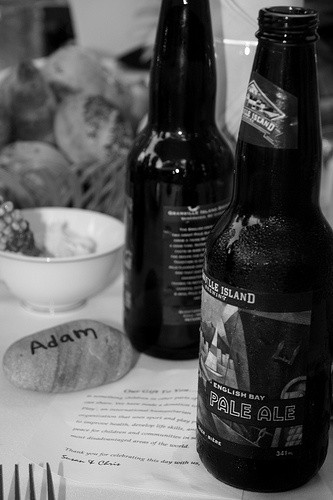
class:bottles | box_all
[197,5,333,494]
[125,0,233,360]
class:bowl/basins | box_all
[0,207,126,313]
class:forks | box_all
[0,462,55,500]
